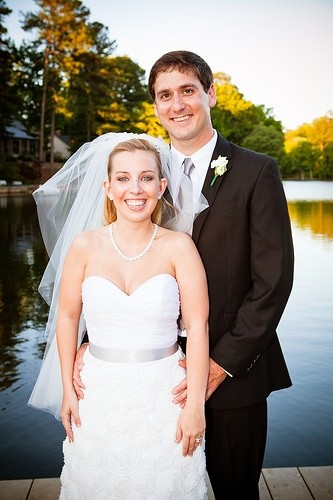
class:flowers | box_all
[211,155,228,186]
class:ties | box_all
[177,157,194,238]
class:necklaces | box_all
[110,222,158,261]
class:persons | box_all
[27,133,208,500]
[72,51,295,500]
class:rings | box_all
[196,438,201,443]
